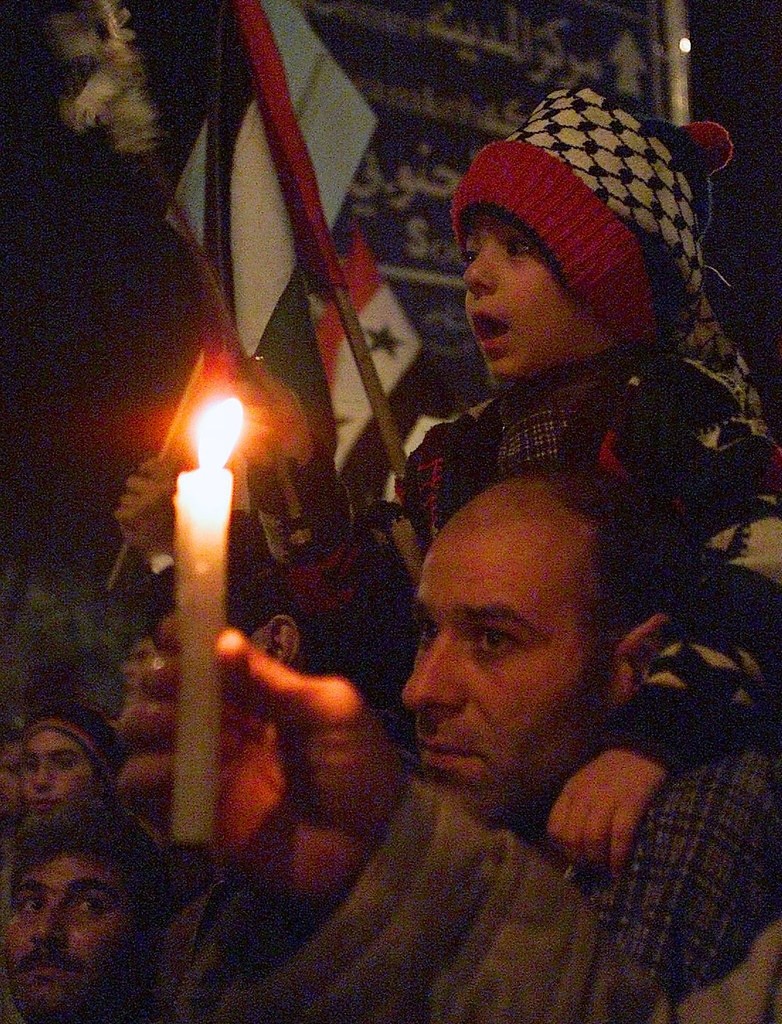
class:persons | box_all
[0,88,782,1024]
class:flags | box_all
[163,0,424,479]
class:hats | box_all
[25,699,111,766]
[452,88,732,337]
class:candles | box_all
[170,398,243,847]
